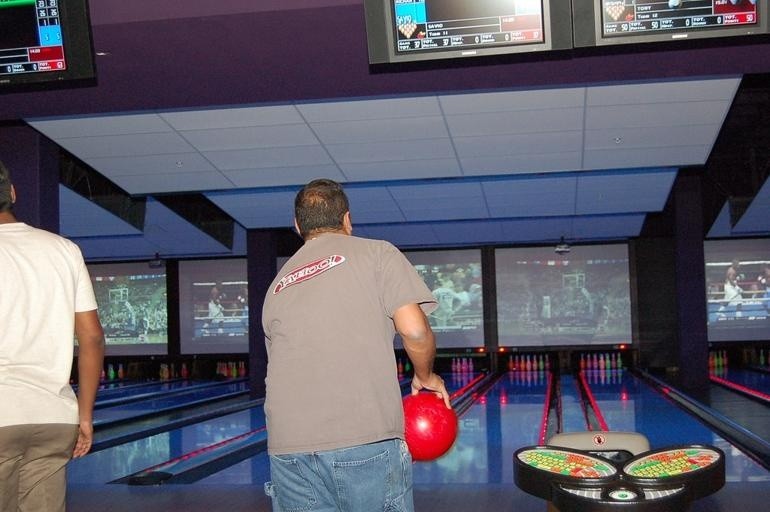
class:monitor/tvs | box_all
[1,0,99,93]
[363,0,572,65]
[572,0,770,49]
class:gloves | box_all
[736,274,745,281]
[219,293,227,300]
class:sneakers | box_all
[716,311,726,317]
[201,328,209,333]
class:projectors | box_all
[555,244,570,255]
[148,260,166,268]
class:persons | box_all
[416,263,482,326]
[0,160,106,511]
[717,260,743,319]
[706,271,768,299]
[92,272,249,341]
[260,177,452,512]
[495,273,632,332]
